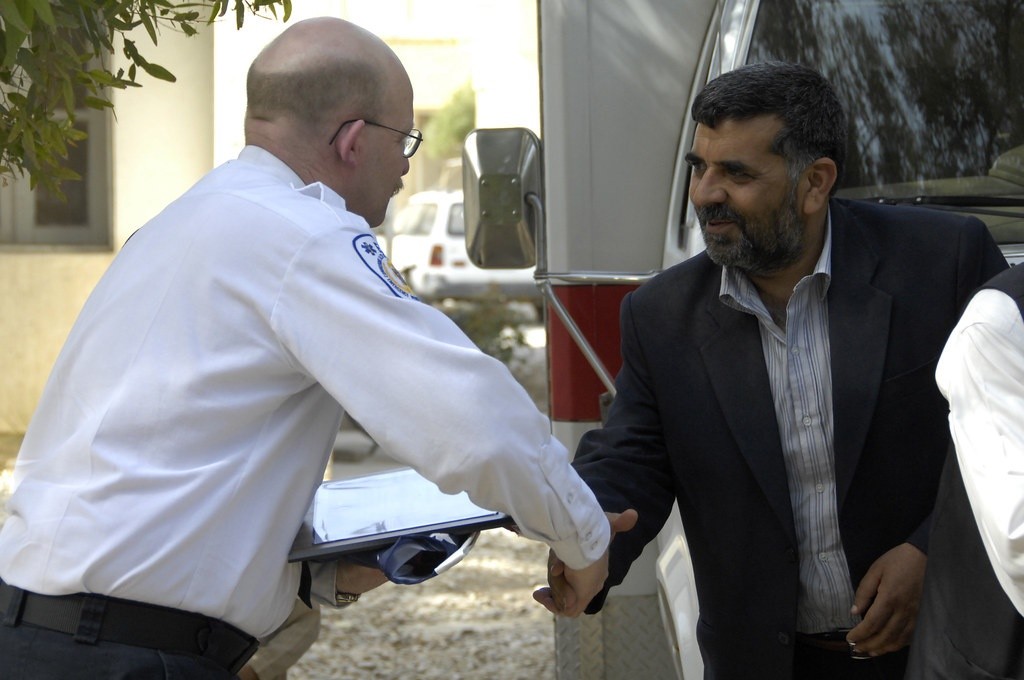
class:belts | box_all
[800,631,876,659]
[1,586,259,675]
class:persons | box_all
[906,261,1024,680]
[0,17,610,680]
[532,64,1011,680]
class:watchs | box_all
[334,588,363,604]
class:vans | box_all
[391,189,544,324]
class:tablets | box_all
[287,465,507,564]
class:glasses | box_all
[329,115,424,158]
